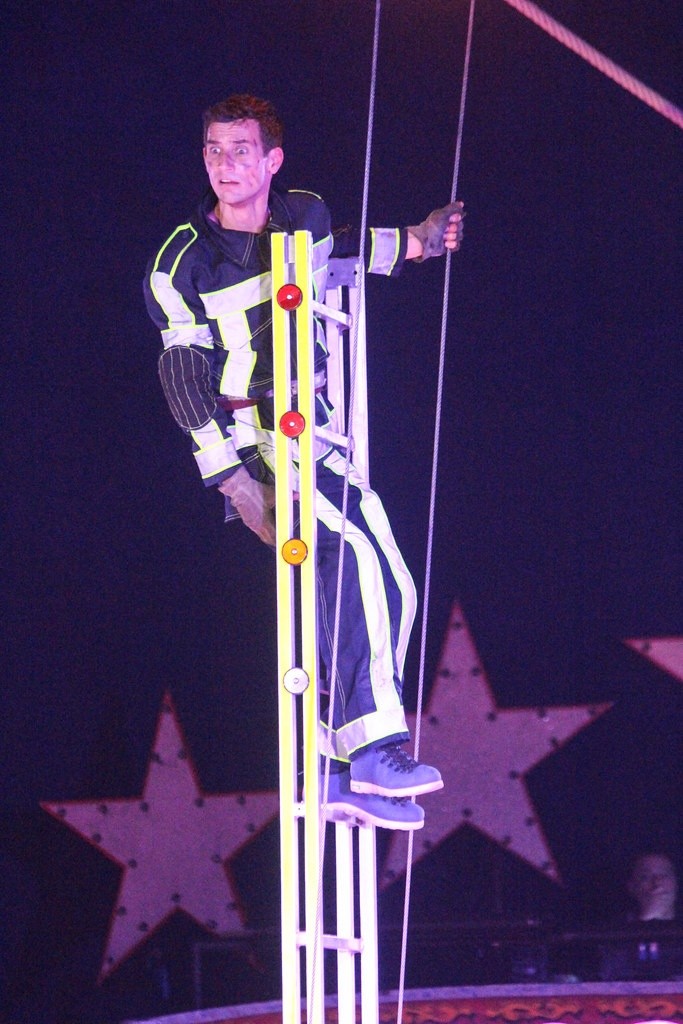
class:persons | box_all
[141,93,464,831]
[613,848,683,981]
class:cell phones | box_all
[653,872,672,893]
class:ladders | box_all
[270,229,380,1024]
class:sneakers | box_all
[350,743,444,798]
[302,765,425,830]
